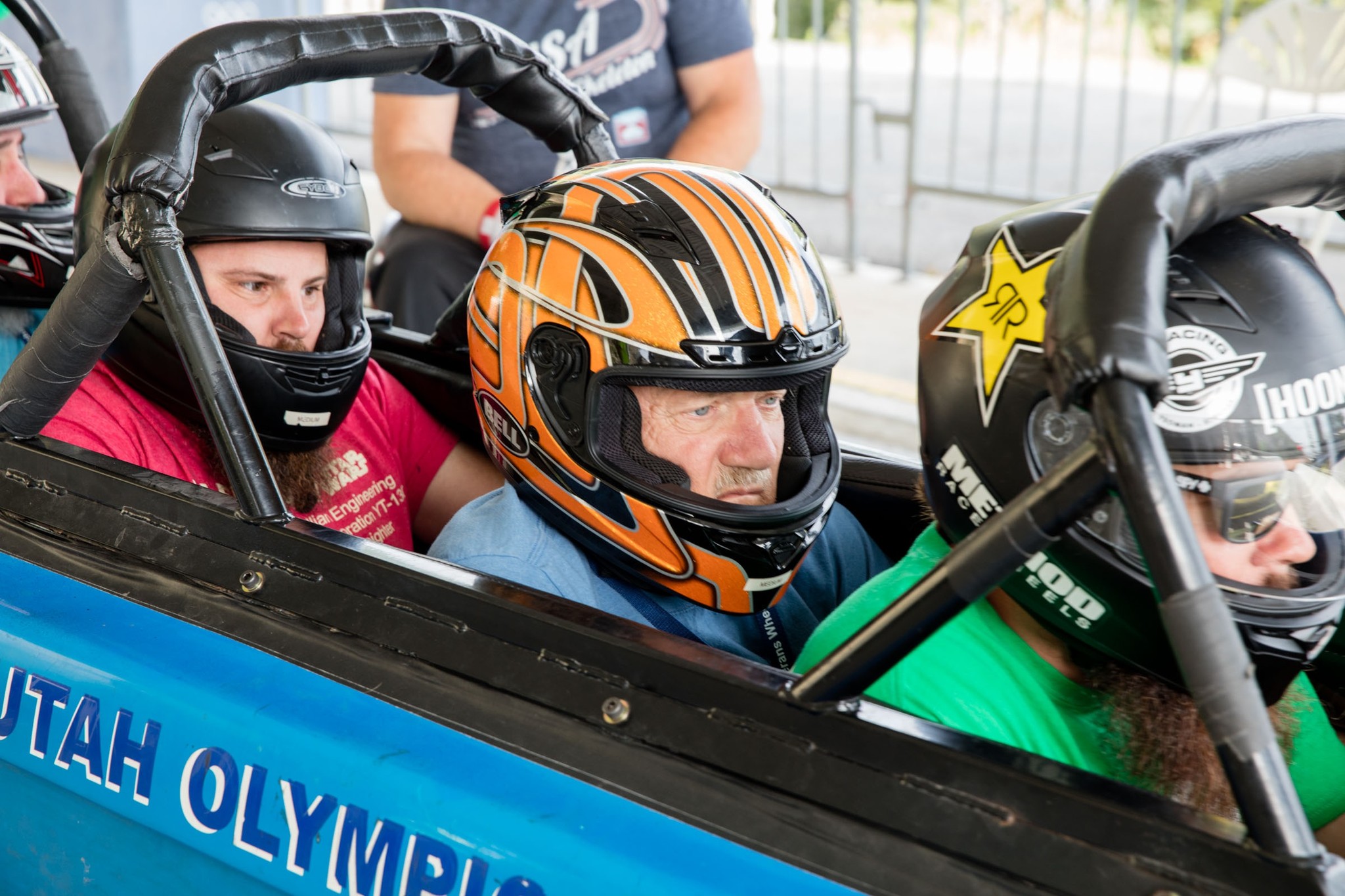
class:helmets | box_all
[70,94,374,451]
[466,158,849,611]
[2,35,80,308]
[916,183,1345,706]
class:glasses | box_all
[1171,449,1343,546]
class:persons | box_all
[0,34,1345,856]
[365,0,766,333]
